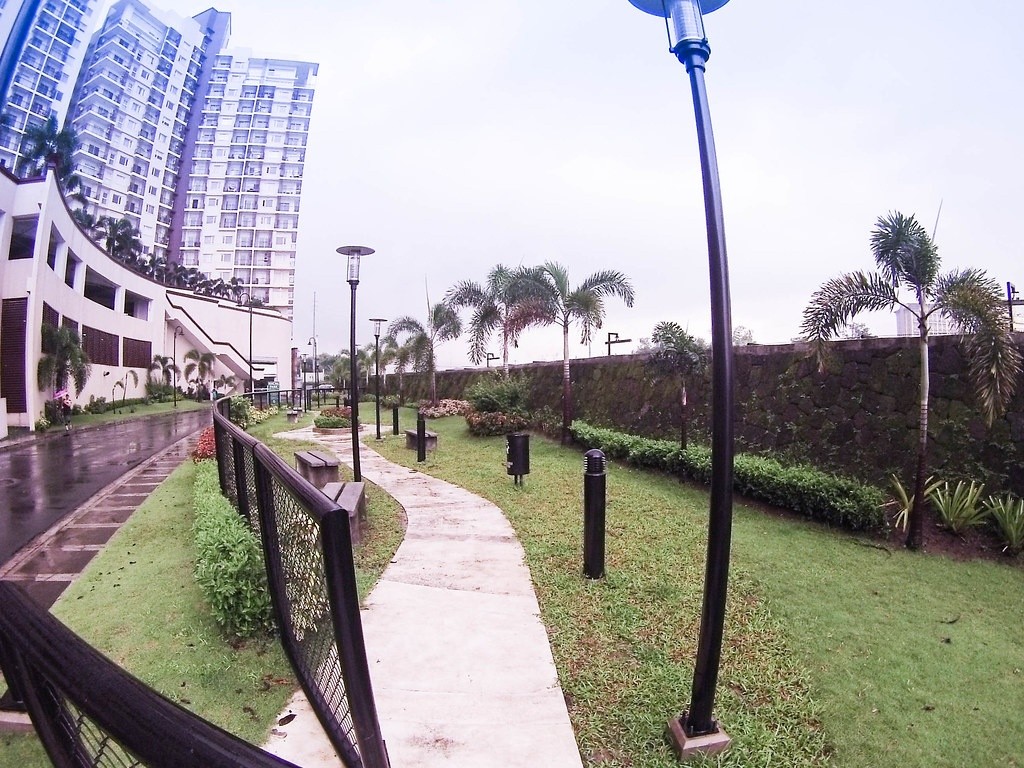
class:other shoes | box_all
[69,424,72,429]
[65,426,69,431]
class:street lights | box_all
[368,318,388,441]
[1001,282,1024,332]
[307,337,317,389]
[235,292,253,393]
[300,353,309,388]
[605,332,632,355]
[173,326,184,406]
[487,352,500,367]
[625,0,737,758]
[335,246,375,483]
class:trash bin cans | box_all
[505,431,531,476]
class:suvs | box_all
[318,385,334,392]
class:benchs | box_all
[293,406,302,416]
[322,482,367,543]
[286,410,298,422]
[293,450,339,489]
[405,428,438,450]
